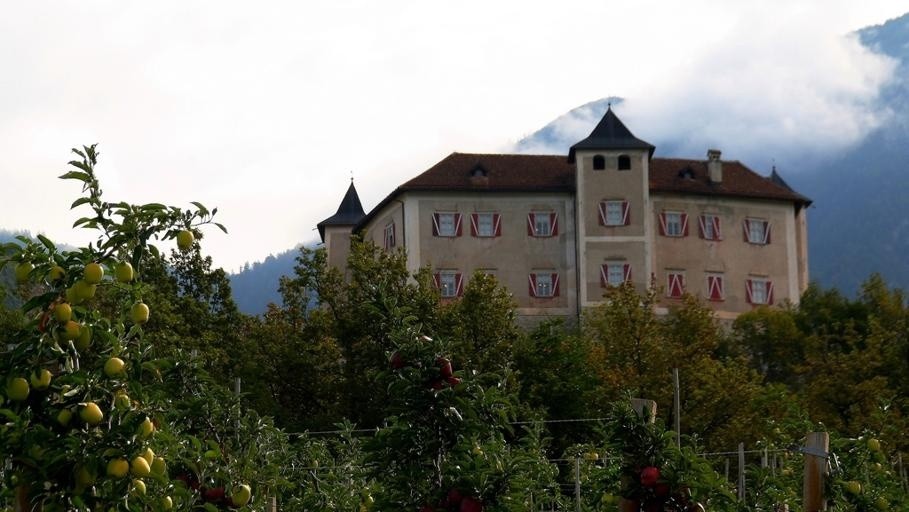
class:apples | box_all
[5,230,889,510]
